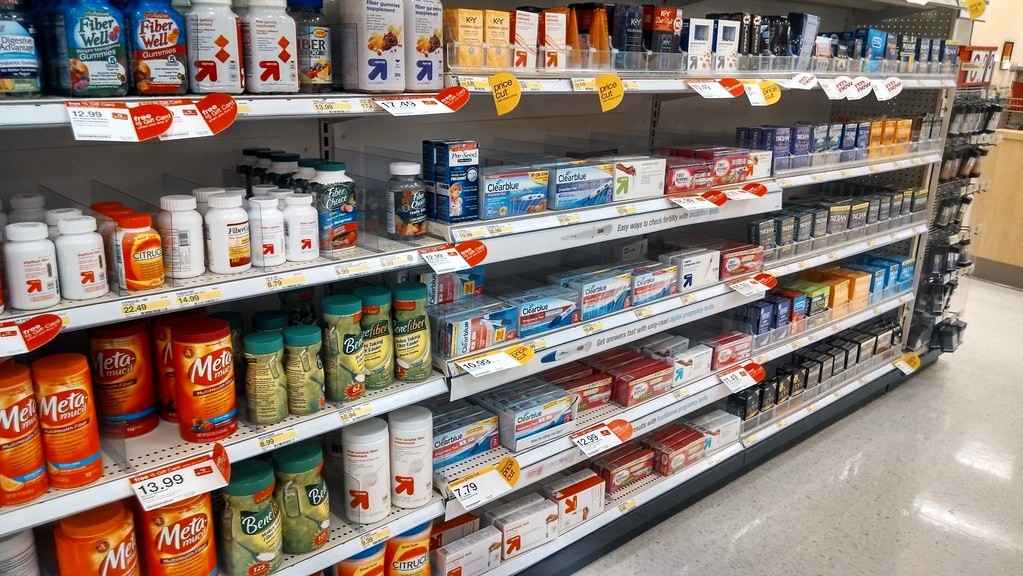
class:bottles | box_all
[89,201,166,292]
[0,524,39,576]
[156,146,359,279]
[241,278,431,505]
[341,404,433,523]
[0,194,108,310]
[220,441,331,576]
[385,161,428,241]
[333,519,433,576]
[0,0,444,101]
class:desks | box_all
[967,129,1023,291]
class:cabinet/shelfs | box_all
[0,0,1009,576]
